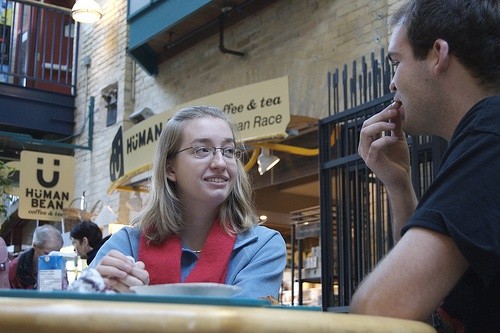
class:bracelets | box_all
[0,253,14,272]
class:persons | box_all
[0,218,115,288]
[61,105,290,303]
[351,0,500,332]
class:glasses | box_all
[173,145,242,160]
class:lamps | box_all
[257,147,280,175]
[70,0,103,24]
[125,191,143,213]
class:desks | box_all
[0,296,436,333]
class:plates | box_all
[129,283,241,297]
